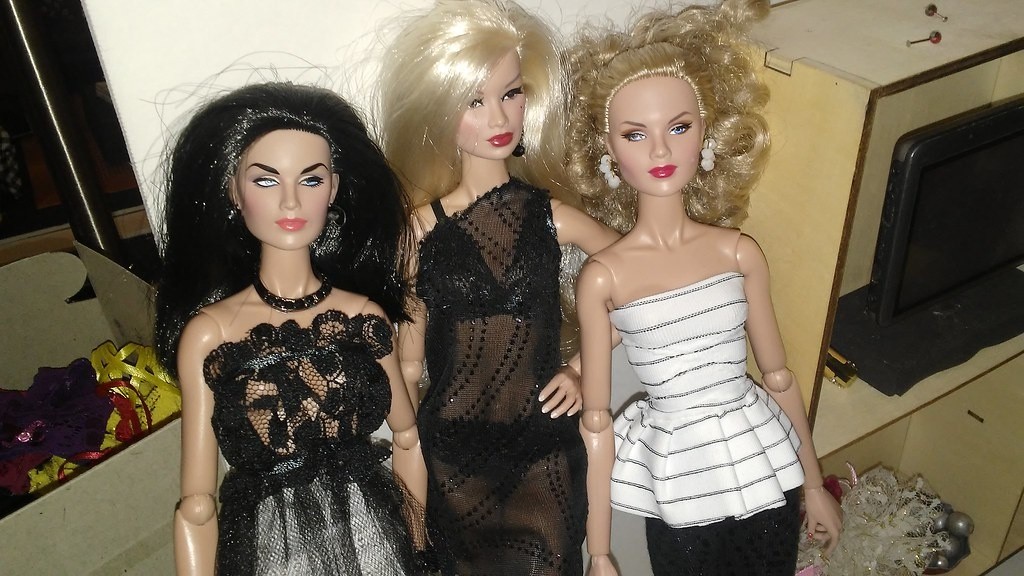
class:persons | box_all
[371,0,624,576]
[542,0,844,576]
[152,83,427,576]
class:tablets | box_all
[868,94,1024,327]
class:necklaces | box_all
[252,271,332,312]
